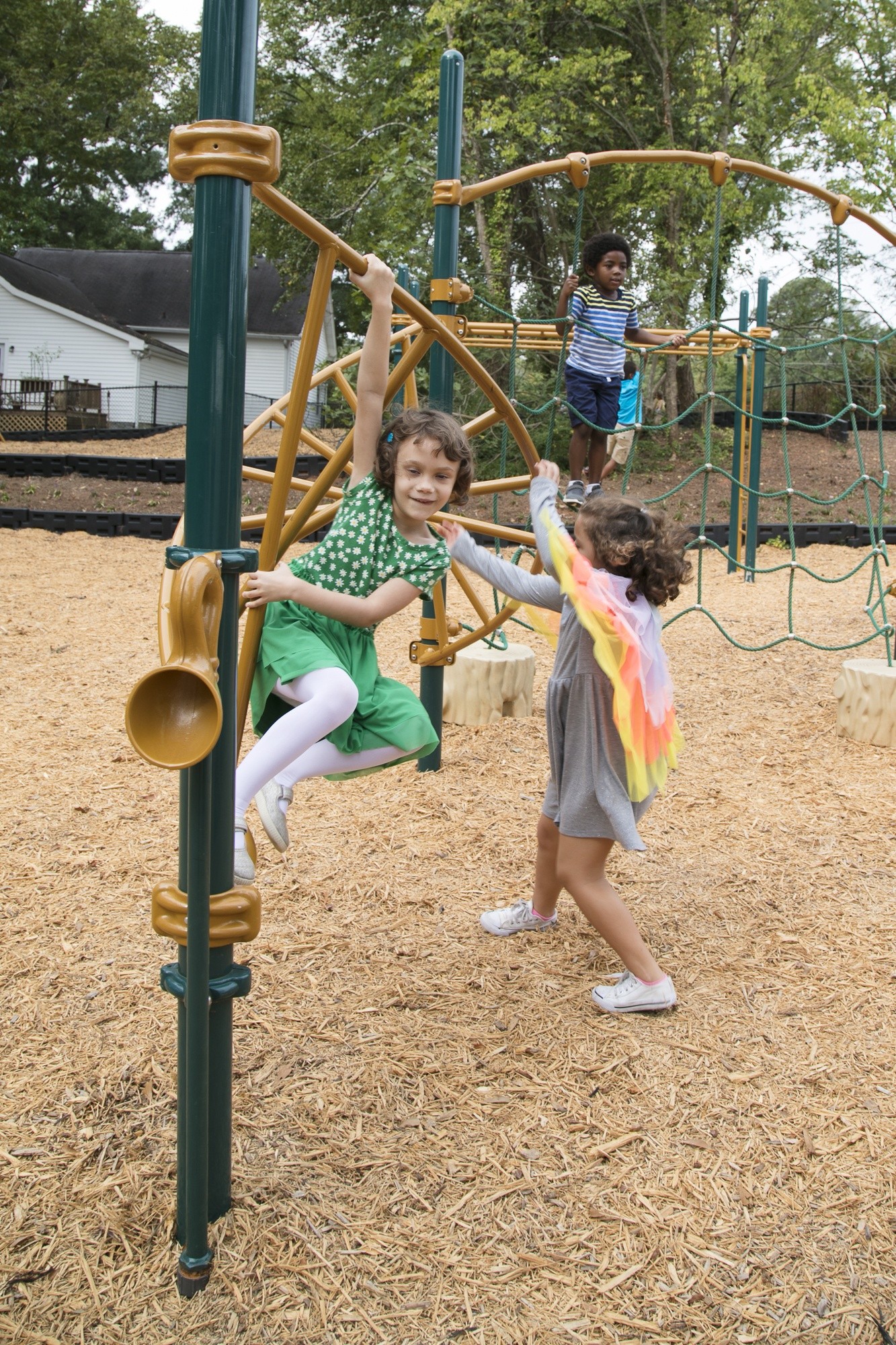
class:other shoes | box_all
[253,779,294,852]
[234,820,256,885]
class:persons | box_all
[555,231,690,501]
[230,254,472,885]
[584,349,648,485]
[649,388,668,440]
[435,457,694,1015]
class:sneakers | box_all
[592,968,676,1012]
[586,485,605,500]
[479,898,558,935]
[563,482,586,506]
